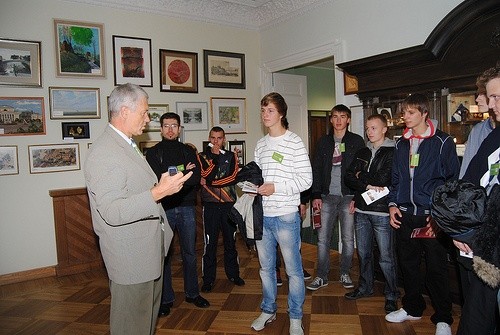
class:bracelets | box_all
[389,205,397,211]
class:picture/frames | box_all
[344,70,358,95]
[0,18,247,175]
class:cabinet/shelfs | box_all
[363,89,500,166]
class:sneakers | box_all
[435,322,452,335]
[306,276,328,290]
[303,268,312,280]
[339,273,355,288]
[344,288,374,299]
[250,311,276,331]
[277,278,283,286]
[385,308,422,323]
[201,282,216,293]
[230,276,245,286]
[289,319,304,335]
[384,300,398,314]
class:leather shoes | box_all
[185,294,210,308]
[157,301,174,317]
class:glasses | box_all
[162,124,179,128]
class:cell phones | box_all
[168,166,177,176]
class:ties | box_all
[132,141,155,176]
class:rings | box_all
[190,167,192,169]
[303,212,305,215]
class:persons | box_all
[75,126,85,136]
[457,67,497,302]
[306,104,364,290]
[250,92,313,335]
[344,114,398,313]
[85,83,193,335]
[385,93,461,335]
[450,71,500,335]
[276,117,313,286]
[146,112,210,317]
[463,101,469,110]
[196,126,245,292]
[234,146,241,163]
